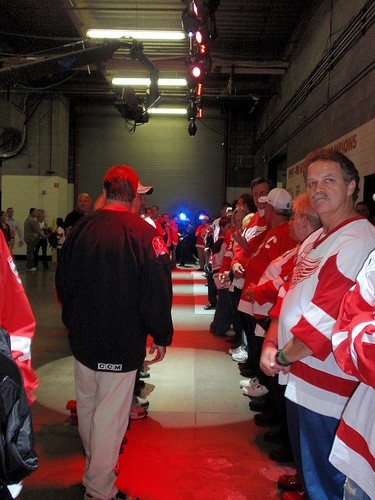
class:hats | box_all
[137,182,154,197]
[257,188,292,209]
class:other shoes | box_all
[204,304,214,310]
[122,437,128,445]
[248,392,279,412]
[225,330,236,336]
[135,380,145,389]
[129,405,148,419]
[253,411,286,427]
[133,387,141,396]
[82,444,125,456]
[139,372,150,378]
[27,267,37,272]
[255,430,290,445]
[277,474,302,491]
[269,447,293,462]
[229,346,246,355]
[232,349,248,362]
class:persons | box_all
[24,208,50,272]
[195,149,375,500]
[0,207,24,250]
[55,218,65,267]
[54,165,178,500]
[65,193,91,238]
[178,219,194,267]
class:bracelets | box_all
[278,348,292,367]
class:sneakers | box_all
[239,377,259,387]
[242,384,269,398]
[132,395,150,408]
[83,490,139,500]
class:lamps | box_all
[180,0,222,138]
